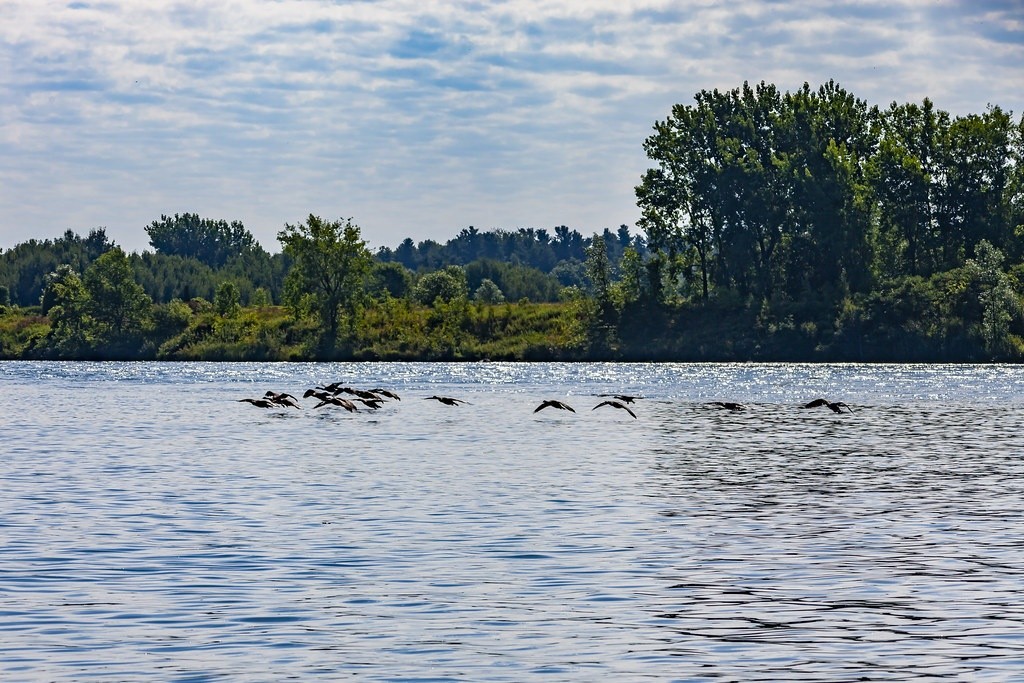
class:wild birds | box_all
[804,398,854,413]
[423,395,474,407]
[591,400,638,420]
[236,381,402,413]
[597,393,644,405]
[532,399,577,415]
[705,400,748,409]
